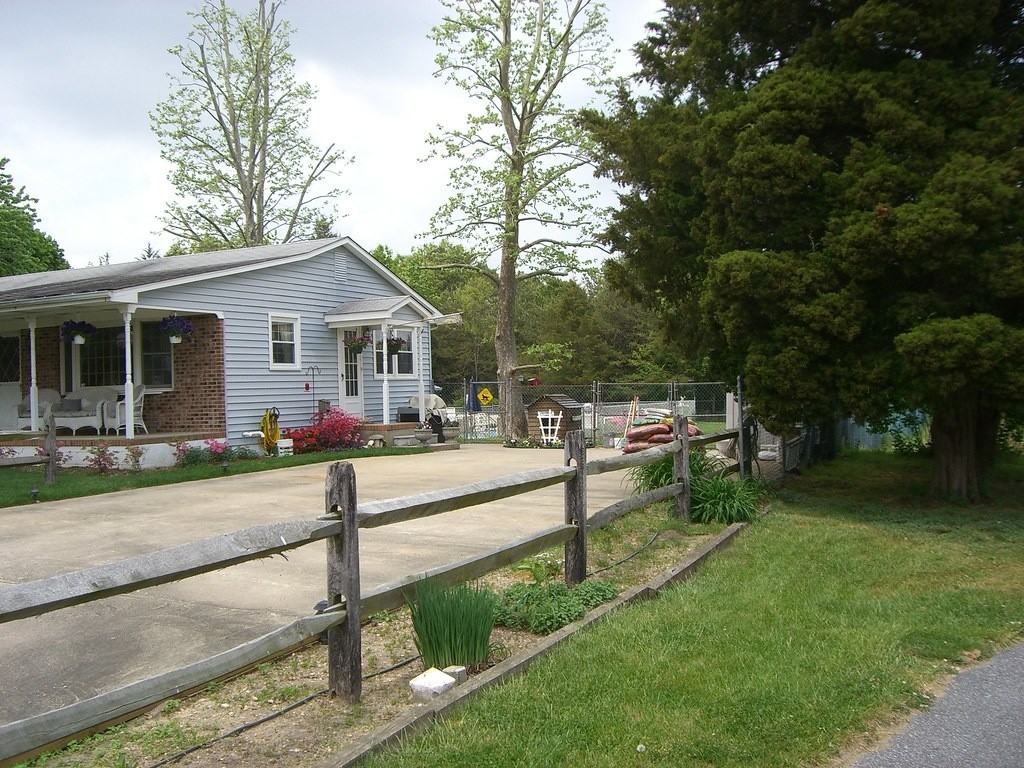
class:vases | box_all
[414,428,432,445]
[169,334,183,344]
[73,334,85,344]
[353,347,361,353]
[389,349,398,354]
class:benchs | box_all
[44,386,118,434]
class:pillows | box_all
[61,398,82,410]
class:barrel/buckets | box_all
[277,439,293,456]
[614,438,622,448]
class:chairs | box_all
[14,388,62,429]
[103,383,148,435]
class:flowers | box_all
[159,313,195,344]
[415,421,432,429]
[61,319,100,343]
[377,336,407,354]
[342,335,372,351]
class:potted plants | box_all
[442,418,461,443]
